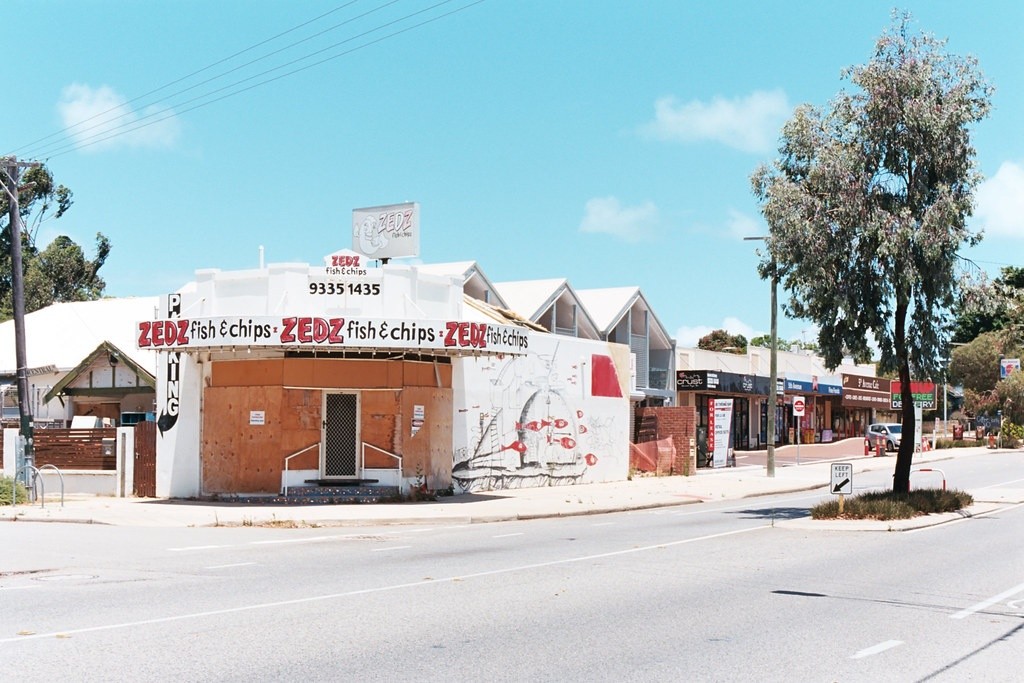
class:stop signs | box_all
[793,395,805,416]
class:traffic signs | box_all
[831,463,852,495]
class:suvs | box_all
[866,422,903,454]
